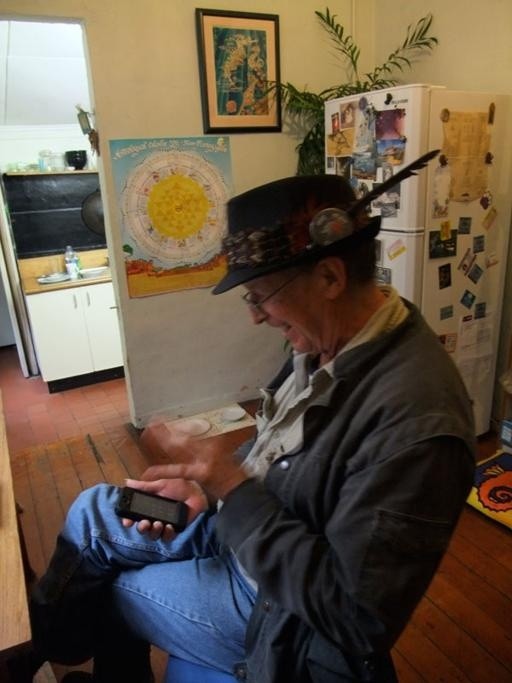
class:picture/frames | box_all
[193,6,283,135]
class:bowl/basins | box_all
[65,150,86,170]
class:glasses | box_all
[242,270,303,314]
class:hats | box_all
[211,174,381,294]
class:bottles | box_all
[64,245,80,282]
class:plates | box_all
[220,403,245,423]
[175,419,212,438]
[37,274,68,284]
[80,266,109,278]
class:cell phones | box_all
[115,487,189,533]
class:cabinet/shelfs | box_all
[18,265,124,394]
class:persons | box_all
[1,172,475,682]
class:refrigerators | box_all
[324,84,512,440]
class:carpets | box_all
[464,447,512,531]
